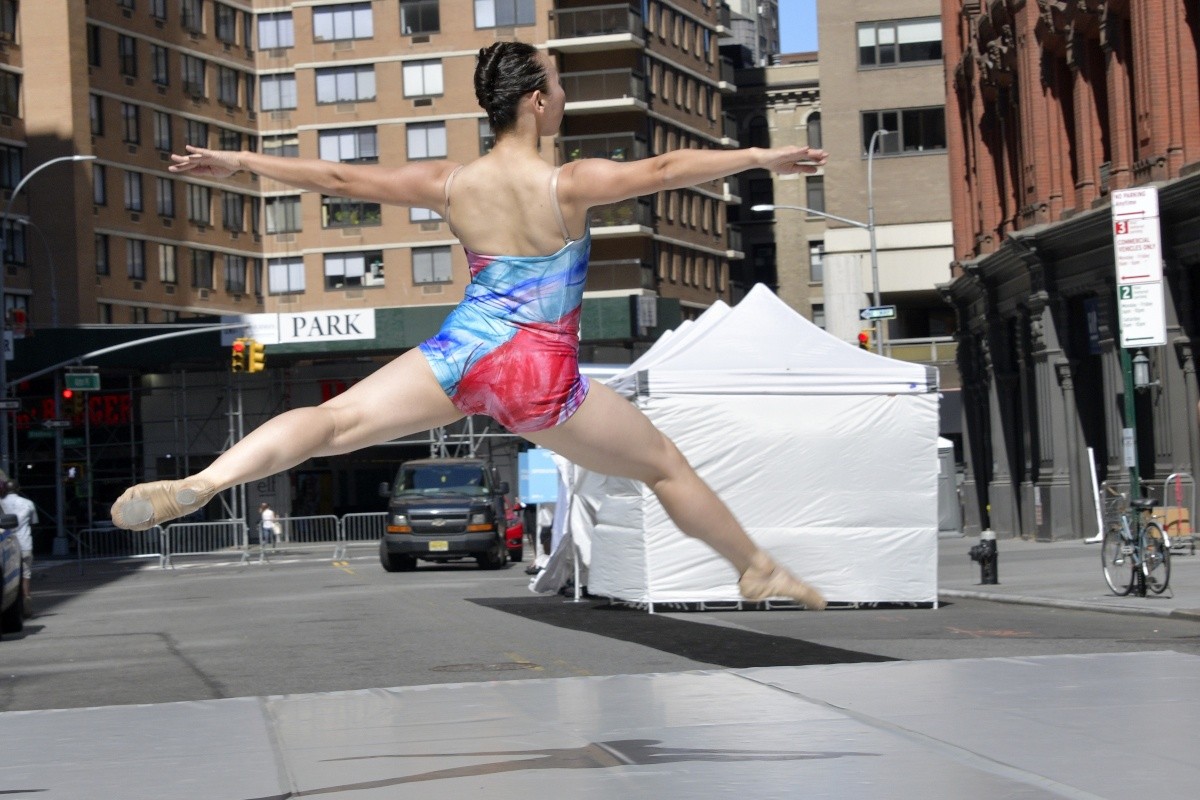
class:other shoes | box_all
[24,595,34,618]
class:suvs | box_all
[379,457,511,572]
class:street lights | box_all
[0,153,98,476]
[750,129,890,357]
[17,218,69,555]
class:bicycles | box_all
[1098,477,1175,599]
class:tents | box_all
[523,284,944,614]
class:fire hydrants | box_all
[967,527,1000,585]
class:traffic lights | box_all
[858,331,872,353]
[231,340,245,373]
[73,391,85,414]
[248,342,265,374]
[60,387,75,417]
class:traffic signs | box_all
[858,305,897,321]
[41,417,74,430]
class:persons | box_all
[111,40,829,611]
[259,503,275,549]
[0,479,40,614]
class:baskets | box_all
[1105,497,1132,523]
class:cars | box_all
[504,495,527,563]
[0,499,25,632]
